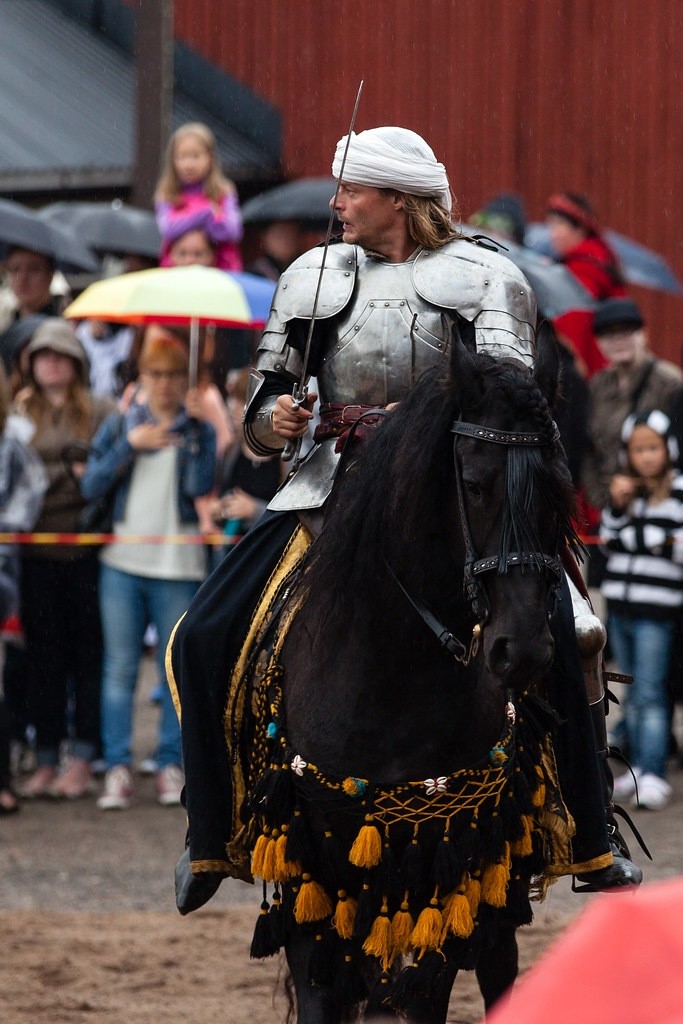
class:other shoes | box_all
[157,762,183,805]
[97,766,135,810]
[0,790,18,815]
[16,769,54,798]
[612,767,642,803]
[631,773,673,812]
[47,761,95,798]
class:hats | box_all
[592,300,644,335]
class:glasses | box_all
[141,367,187,380]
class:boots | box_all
[175,846,223,917]
[575,685,642,894]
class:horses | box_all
[237,323,589,1024]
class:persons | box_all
[161,126,643,892]
[0,126,683,809]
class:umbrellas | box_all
[456,224,598,316]
[0,202,95,271]
[41,201,160,262]
[62,264,279,455]
[240,178,345,220]
[524,223,677,295]
